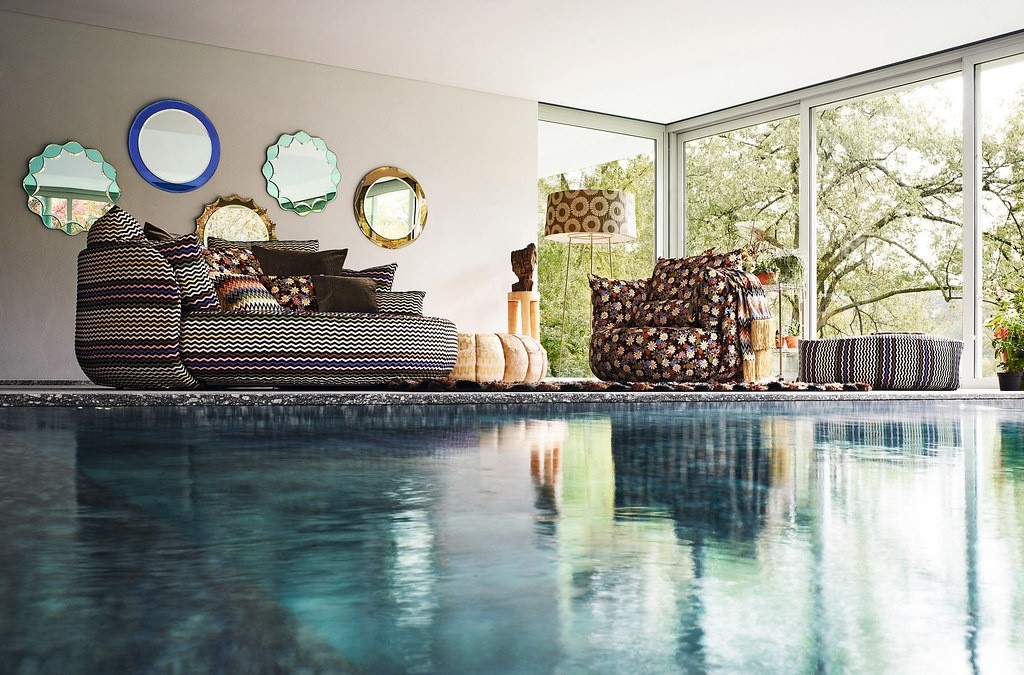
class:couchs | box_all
[586,249,743,383]
[75,247,459,391]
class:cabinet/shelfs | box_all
[760,281,805,381]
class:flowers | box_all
[982,314,1024,372]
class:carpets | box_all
[381,381,873,391]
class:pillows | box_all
[86,205,426,317]
[632,247,716,326]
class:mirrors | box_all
[261,131,342,216]
[23,140,121,236]
[127,99,221,193]
[194,194,277,248]
[353,165,429,249]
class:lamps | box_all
[545,190,638,376]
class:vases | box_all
[998,373,1024,391]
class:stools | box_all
[449,333,547,384]
[796,334,964,392]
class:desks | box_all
[507,291,541,343]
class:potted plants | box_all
[775,322,800,349]
[753,253,804,285]
